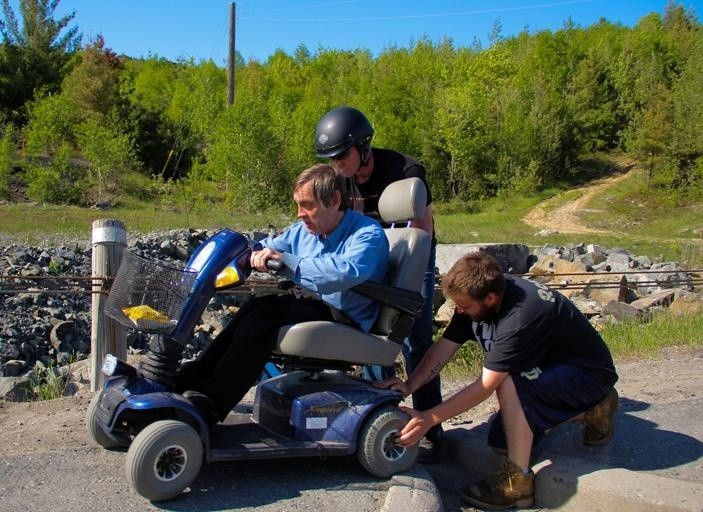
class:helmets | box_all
[314,107,375,159]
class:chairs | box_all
[270,176,433,372]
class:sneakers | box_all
[459,456,536,512]
[583,386,620,447]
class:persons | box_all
[311,105,447,466]
[374,250,621,512]
[144,163,391,433]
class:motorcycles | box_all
[83,177,436,502]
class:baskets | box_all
[103,247,197,332]
